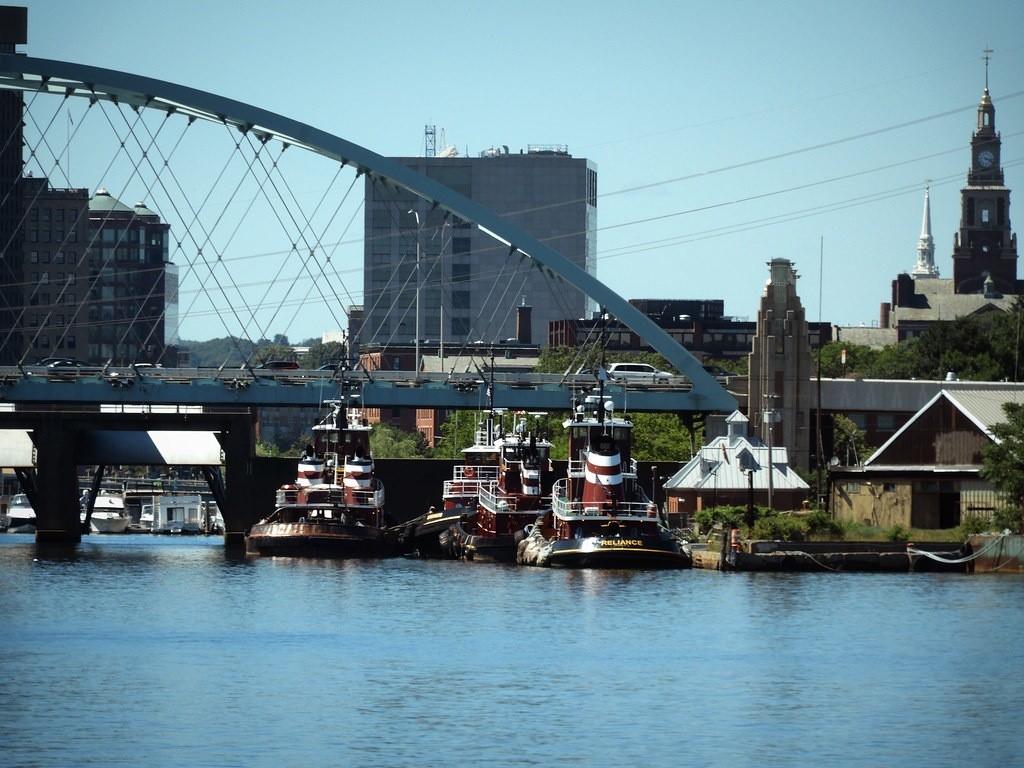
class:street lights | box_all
[440,221,451,372]
[763,411,775,512]
[407,208,421,378]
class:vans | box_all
[253,361,301,370]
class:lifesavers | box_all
[438,529,471,561]
[464,467,474,477]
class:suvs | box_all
[109,362,169,377]
[598,362,675,384]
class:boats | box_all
[78,487,132,534]
[140,495,225,537]
[436,351,558,563]
[513,311,692,569]
[5,493,38,533]
[242,376,390,558]
[128,523,151,534]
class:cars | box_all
[566,368,628,385]
[315,363,352,372]
[702,364,738,376]
[25,357,103,376]
